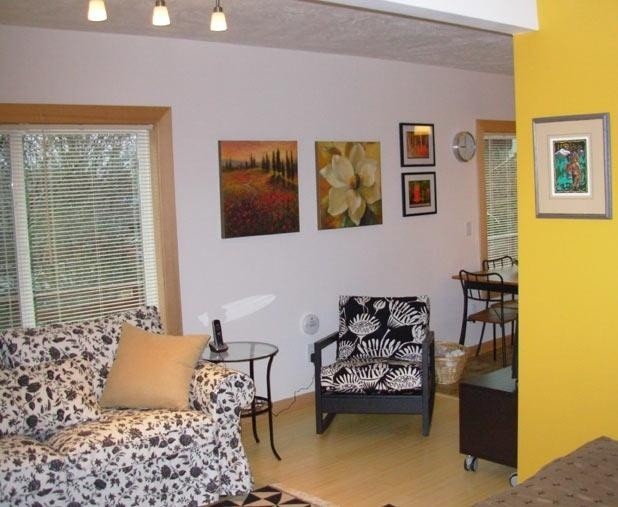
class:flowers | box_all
[317,142,382,227]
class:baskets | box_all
[435,341,466,385]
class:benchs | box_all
[466,430,618,506]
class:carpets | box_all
[194,479,338,506]
[427,340,519,400]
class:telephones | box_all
[209,320,228,353]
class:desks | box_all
[190,336,285,460]
[450,264,519,379]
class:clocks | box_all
[451,129,478,162]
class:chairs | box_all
[306,292,440,437]
[473,254,514,362]
[456,267,519,369]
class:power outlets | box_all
[304,341,322,364]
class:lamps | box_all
[86,0,108,22]
[209,0,229,32]
[151,0,171,26]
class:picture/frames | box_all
[401,170,437,218]
[527,108,614,221]
[398,119,436,168]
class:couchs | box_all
[0,302,256,505]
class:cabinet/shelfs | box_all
[452,362,519,491]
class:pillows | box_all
[94,316,212,413]
[5,347,106,444]
[1,362,32,437]
